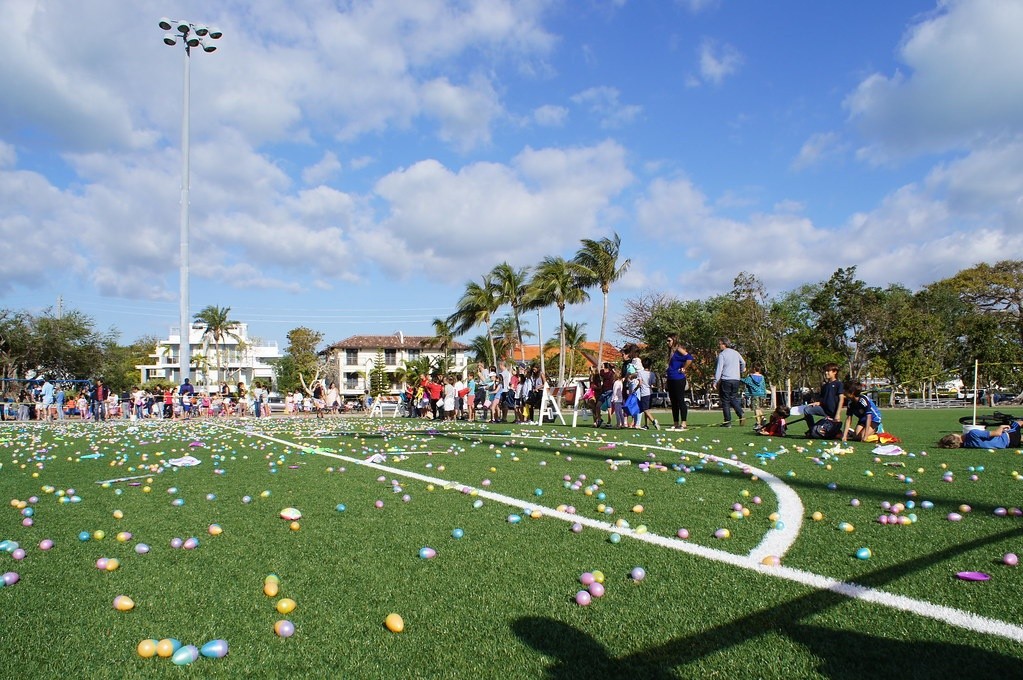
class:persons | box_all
[284,382,341,418]
[580,343,661,431]
[935,421,1023,449]
[804,363,844,438]
[712,337,746,427]
[757,406,790,436]
[842,380,884,441]
[666,334,692,432]
[402,360,546,423]
[0,376,270,422]
[740,362,767,429]
[363,390,372,416]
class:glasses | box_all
[667,340,673,343]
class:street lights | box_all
[160,18,222,385]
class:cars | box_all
[375,400,398,410]
[268,396,286,409]
[696,393,721,408]
[957,388,986,401]
[652,393,691,407]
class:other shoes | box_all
[674,425,688,431]
[753,423,761,429]
[593,419,650,430]
[720,422,731,428]
[466,417,509,424]
[653,419,660,429]
[761,417,767,423]
[665,424,678,431]
[513,419,537,426]
[739,417,745,426]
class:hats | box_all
[488,372,497,376]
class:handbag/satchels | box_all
[624,392,643,418]
[807,418,843,439]
[436,398,444,407]
